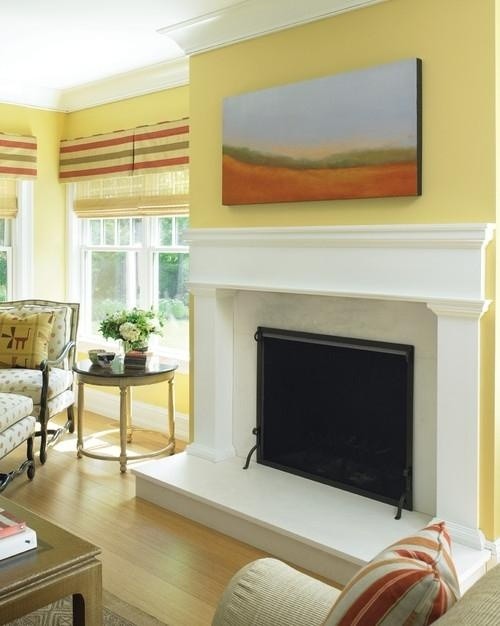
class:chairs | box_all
[0,393,37,481]
[0,299,80,465]
[211,519,499,625]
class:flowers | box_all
[100,305,171,347]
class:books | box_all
[1,505,24,539]
[0,526,38,561]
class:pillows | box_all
[0,311,56,370]
[319,521,464,626]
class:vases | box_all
[124,346,153,370]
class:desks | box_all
[72,357,181,472]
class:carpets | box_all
[1,586,170,626]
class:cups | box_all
[88,349,116,369]
